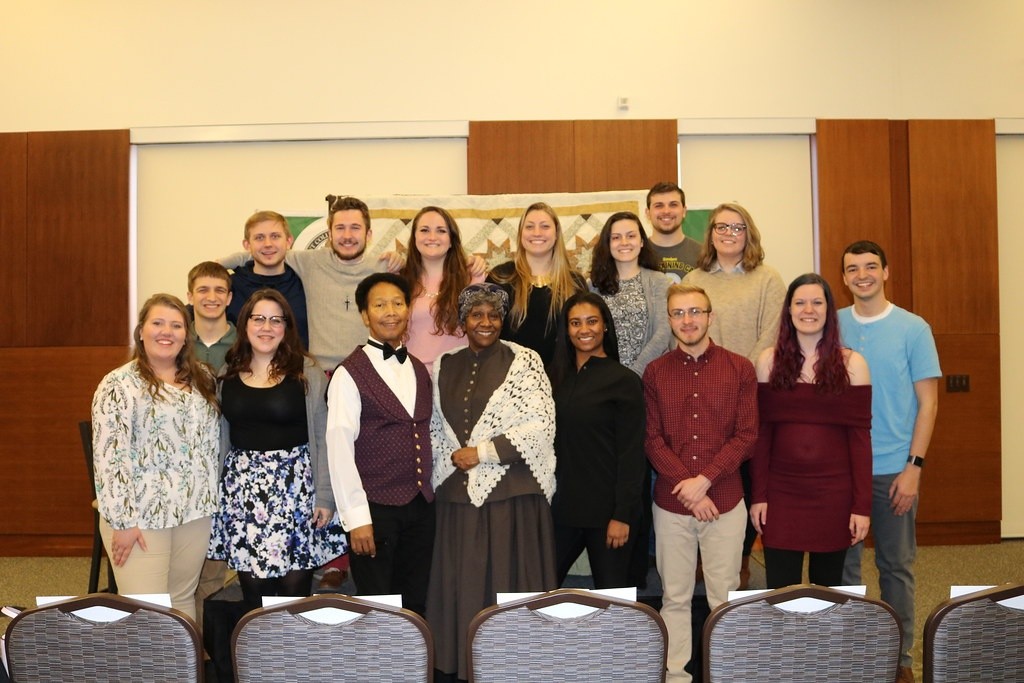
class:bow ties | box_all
[367,339,407,364]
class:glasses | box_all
[711,223,747,237]
[668,306,709,319]
[248,314,287,328]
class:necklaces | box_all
[531,273,551,286]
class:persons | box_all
[547,295,642,585]
[326,272,437,613]
[91,295,226,665]
[184,182,785,588]
[206,292,347,605]
[433,284,558,680]
[837,239,945,682]
[752,273,873,587]
[642,284,758,683]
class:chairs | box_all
[230,594,433,683]
[6,592,203,683]
[923,583,1024,683]
[464,588,669,683]
[702,584,903,683]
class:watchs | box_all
[907,456,925,468]
